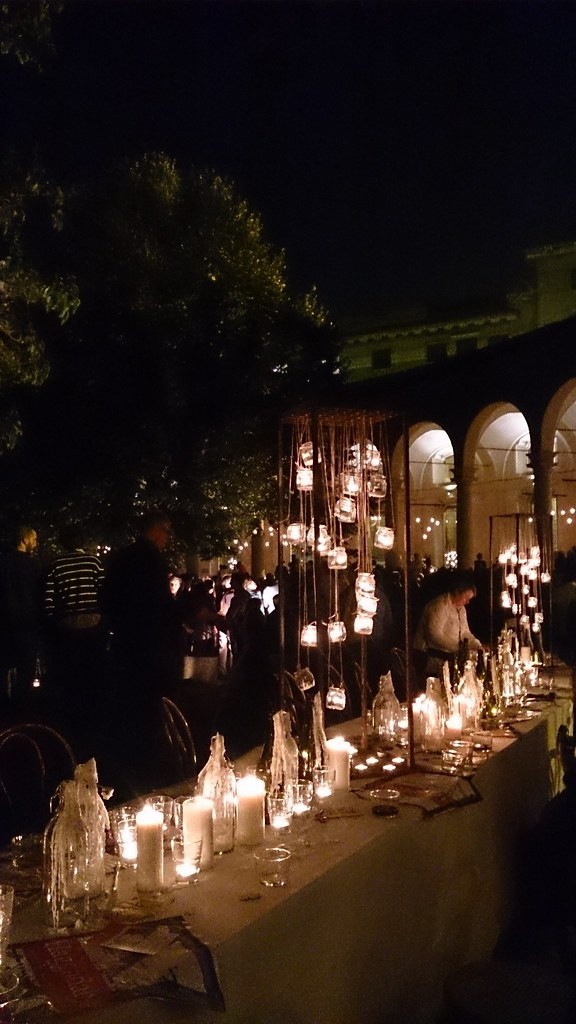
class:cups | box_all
[313,765,335,796]
[453,741,475,765]
[441,750,465,776]
[369,789,400,820]
[472,732,485,751]
[291,779,313,813]
[253,848,292,887]
[108,795,203,876]
[267,792,293,828]
[0,884,14,973]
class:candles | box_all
[325,735,350,791]
[446,718,462,741]
[411,698,422,745]
[236,774,265,846]
[452,693,470,729]
[135,804,164,893]
[520,646,530,662]
[182,796,215,871]
[383,764,396,774]
[365,756,379,768]
[354,763,369,774]
[392,756,405,768]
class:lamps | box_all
[487,512,557,651]
[272,402,407,716]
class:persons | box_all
[0,513,576,789]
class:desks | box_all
[0,644,576,1024]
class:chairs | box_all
[161,696,202,778]
[0,722,77,843]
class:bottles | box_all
[457,661,479,736]
[372,675,400,748]
[502,643,514,666]
[420,678,445,752]
[135,803,164,892]
[237,767,266,846]
[198,735,236,852]
[475,649,486,679]
